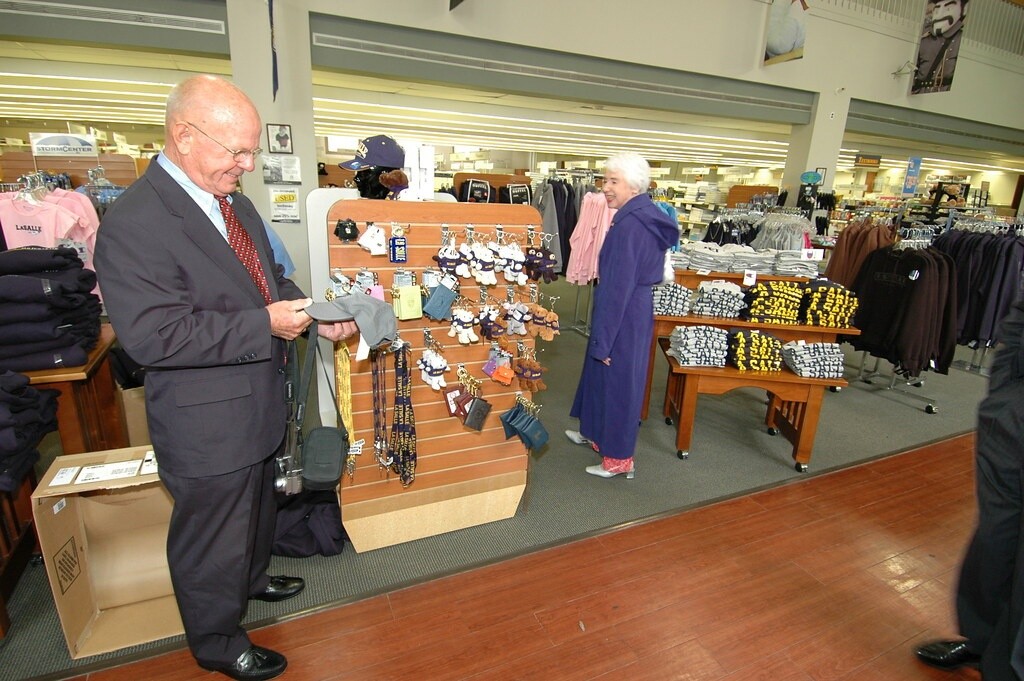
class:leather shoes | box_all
[248,575,305,602]
[918,640,981,669]
[196,645,287,681]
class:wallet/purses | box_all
[454,386,549,450]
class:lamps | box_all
[891,59,918,74]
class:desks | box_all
[22,325,130,458]
[657,338,849,473]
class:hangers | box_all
[853,205,935,252]
[952,212,1024,237]
[12,171,52,208]
[83,167,110,188]
[711,203,818,236]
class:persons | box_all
[337,135,405,199]
[912,272,1024,671]
[94,75,358,681]
[564,154,678,480]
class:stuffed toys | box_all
[417,350,450,390]
[515,358,548,392]
[448,303,560,344]
[433,243,559,287]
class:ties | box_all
[215,195,288,366]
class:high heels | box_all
[586,464,634,479]
[565,429,597,452]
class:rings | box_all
[302,328,306,333]
[343,334,351,338]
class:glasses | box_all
[189,121,263,163]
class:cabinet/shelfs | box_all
[668,199,718,227]
[639,269,862,426]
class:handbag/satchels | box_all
[300,426,347,491]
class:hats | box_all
[303,293,396,350]
[338,134,404,171]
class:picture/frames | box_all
[266,123,293,154]
[814,168,827,186]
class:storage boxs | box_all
[30,445,185,661]
